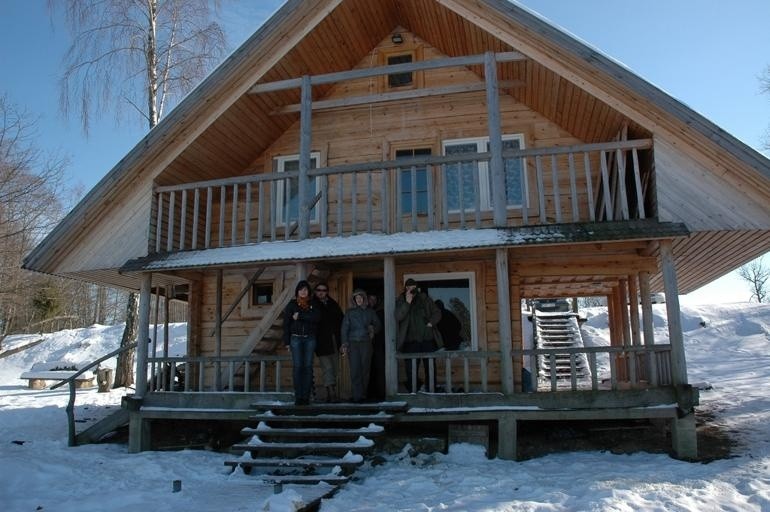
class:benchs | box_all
[20,375,96,390]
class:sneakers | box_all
[295,398,310,406]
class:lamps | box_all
[392,34,403,44]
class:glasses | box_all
[314,289,328,292]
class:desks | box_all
[34,362,97,388]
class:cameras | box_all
[409,288,420,295]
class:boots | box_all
[326,384,338,403]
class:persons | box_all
[284,279,443,407]
[435,300,462,350]
